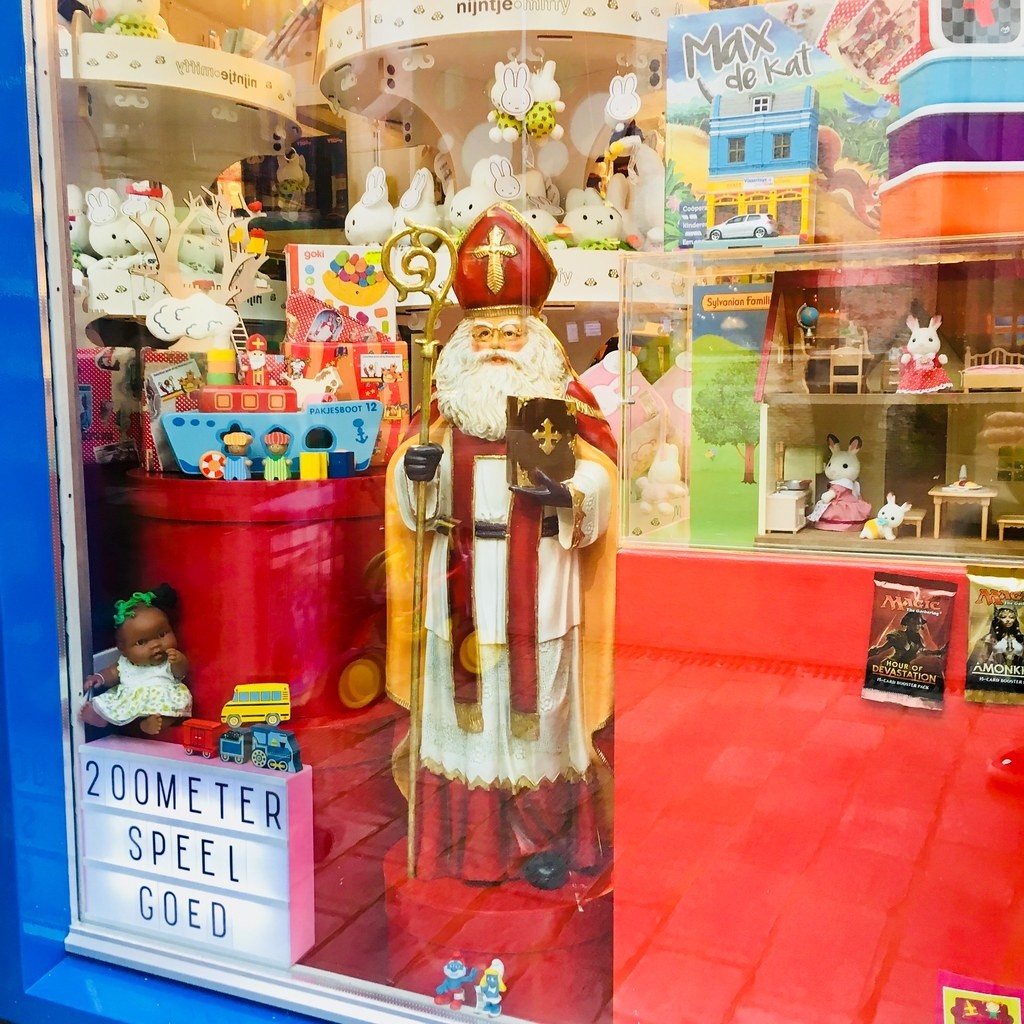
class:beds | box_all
[958,346,1024,393]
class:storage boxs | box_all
[281,342,411,467]
[284,243,397,343]
[74,347,140,465]
[618,0,1024,565]
[127,351,208,474]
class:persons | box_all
[384,200,622,892]
[77,582,194,735]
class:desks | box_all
[928,485,997,542]
[792,314,874,394]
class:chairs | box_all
[829,345,863,394]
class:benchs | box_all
[893,508,927,538]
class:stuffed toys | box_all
[76,0,179,48]
[66,180,273,288]
[806,433,872,532]
[275,153,309,211]
[859,492,913,542]
[895,312,954,395]
[344,60,665,251]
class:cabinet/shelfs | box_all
[317,0,710,416]
[881,359,901,394]
[766,490,813,535]
[58,10,302,348]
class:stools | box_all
[996,515,1024,542]
